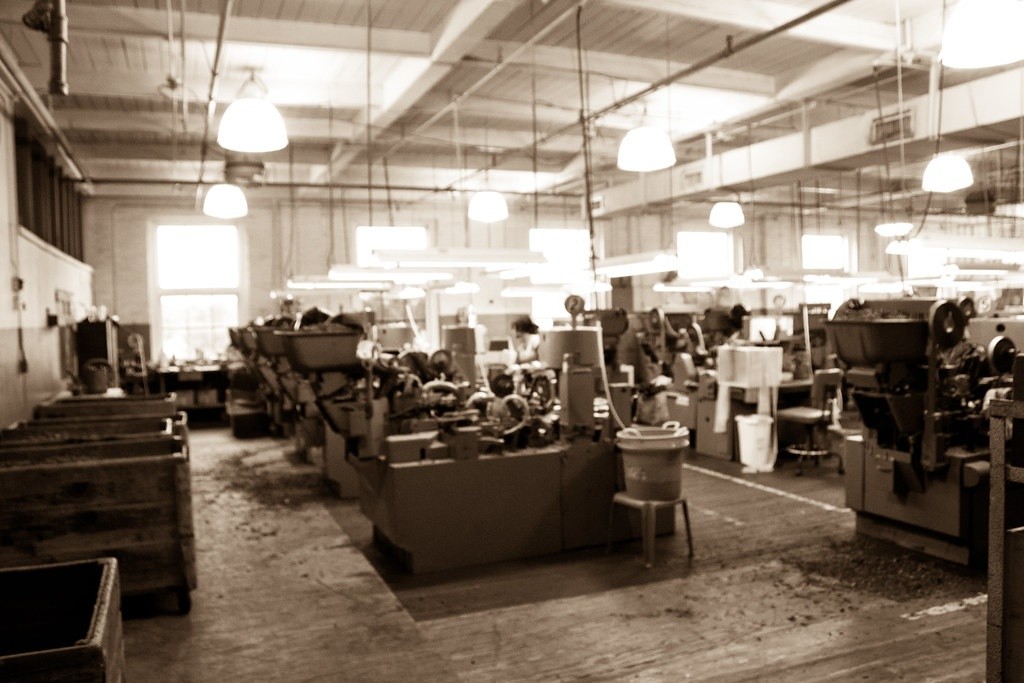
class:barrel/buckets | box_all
[735,413,774,467]
[617,421,691,501]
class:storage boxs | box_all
[717,344,784,388]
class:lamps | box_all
[200,0,976,237]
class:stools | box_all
[605,492,694,567]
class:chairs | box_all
[777,367,845,477]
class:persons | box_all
[510,315,541,364]
